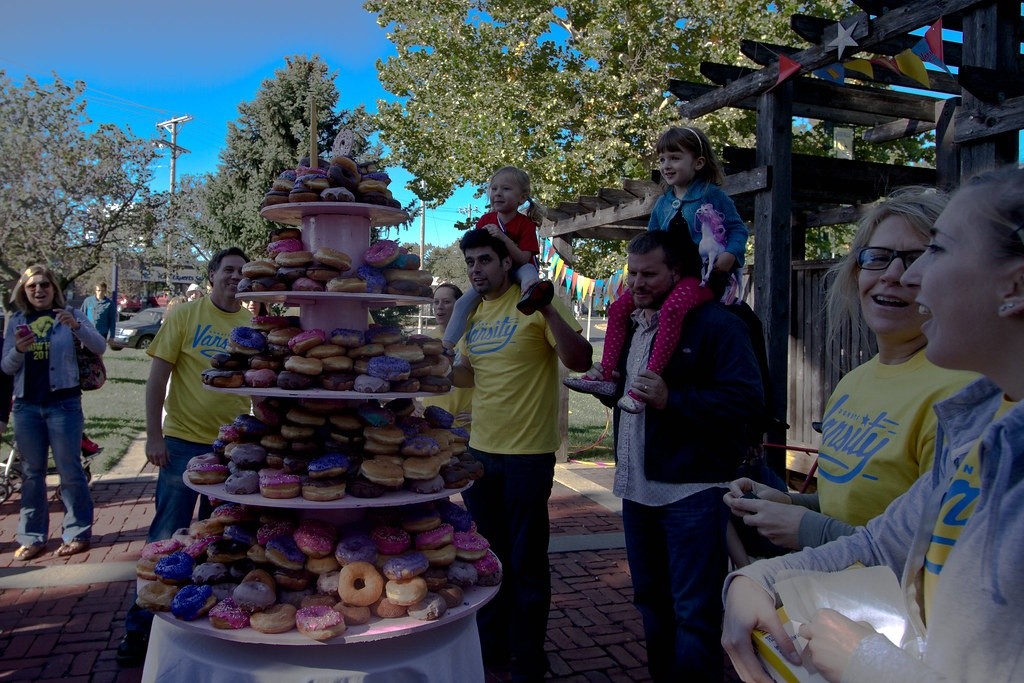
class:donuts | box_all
[136,154,502,640]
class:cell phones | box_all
[16,325,30,338]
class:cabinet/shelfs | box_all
[132,154,502,682]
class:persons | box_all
[719,188,986,558]
[79,281,117,347]
[441,226,595,683]
[240,299,270,318]
[0,266,109,561]
[186,284,206,301]
[586,226,773,683]
[422,282,477,443]
[438,166,555,362]
[558,125,752,417]
[115,247,268,670]
[719,158,1024,683]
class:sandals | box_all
[55,543,91,557]
[14,545,46,561]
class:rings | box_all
[643,384,648,393]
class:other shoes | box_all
[563,377,616,396]
[117,632,148,663]
[517,280,554,316]
[618,394,646,413]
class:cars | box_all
[117,293,142,313]
[146,291,179,307]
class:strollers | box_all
[0,393,105,503]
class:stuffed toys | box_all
[696,204,744,308]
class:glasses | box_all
[855,246,927,271]
[24,282,52,289]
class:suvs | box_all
[107,307,168,351]
